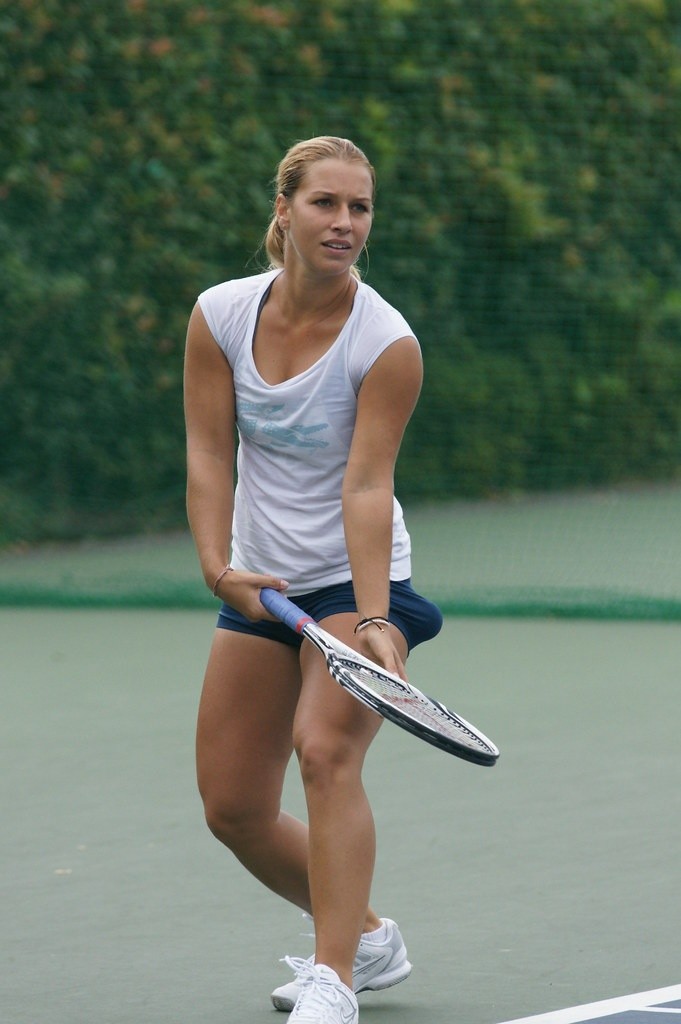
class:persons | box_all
[182,134,431,1024]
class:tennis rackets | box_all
[258,583,503,770]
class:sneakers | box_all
[269,917,412,1024]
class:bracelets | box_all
[354,616,388,633]
[212,566,233,597]
